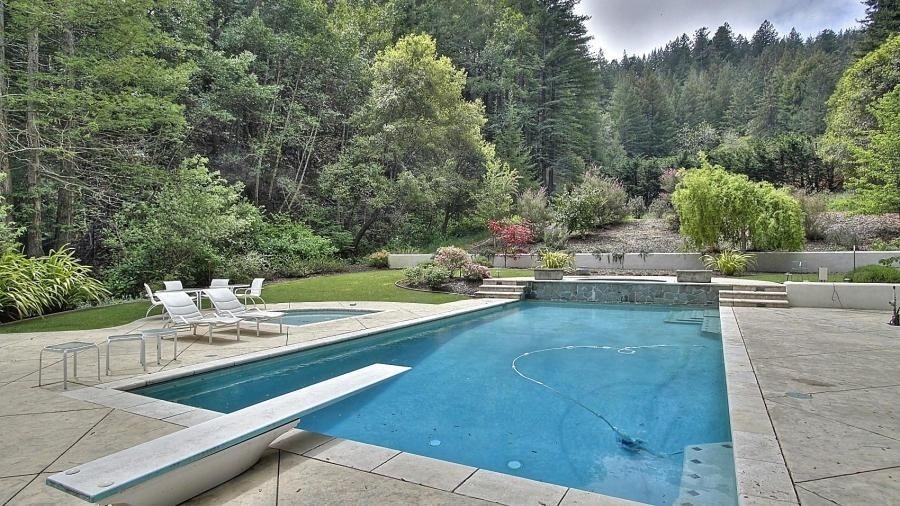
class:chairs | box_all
[144,278,287,344]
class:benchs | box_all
[676,269,712,283]
[534,269,563,279]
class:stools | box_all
[141,329,176,367]
[105,335,150,375]
[39,342,100,389]
